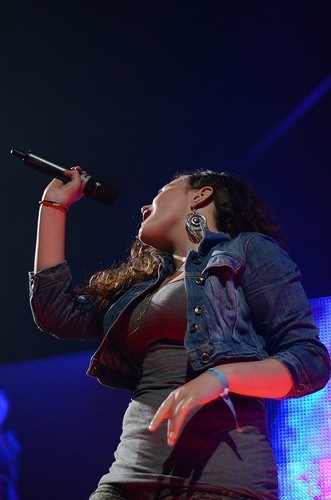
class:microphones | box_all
[10,144,119,205]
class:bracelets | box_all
[38,200,68,213]
[205,368,242,432]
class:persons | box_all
[28,166,331,500]
